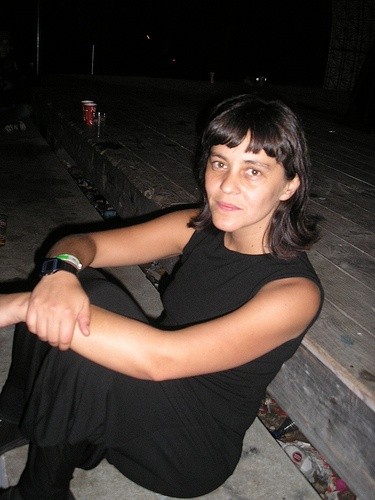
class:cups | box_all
[81,100,94,122]
[83,103,98,126]
[92,112,106,139]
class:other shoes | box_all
[0,420,29,459]
[0,484,20,500]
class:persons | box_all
[0,95,328,500]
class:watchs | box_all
[39,259,80,280]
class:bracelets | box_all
[50,254,83,270]
